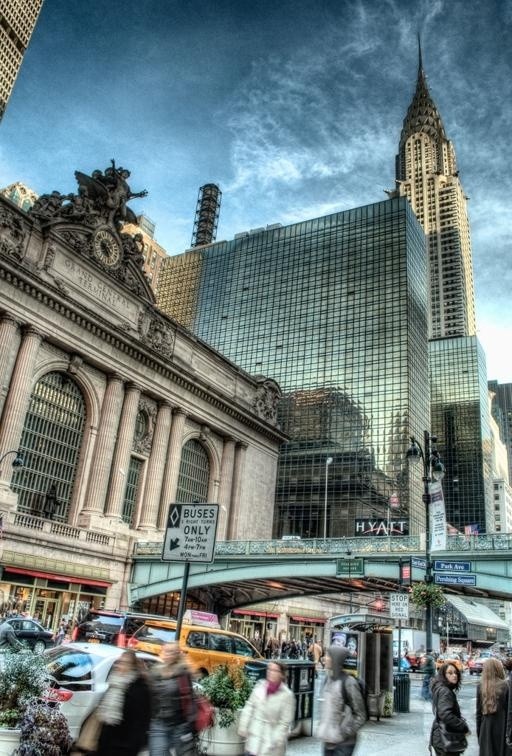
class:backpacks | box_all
[341,673,369,723]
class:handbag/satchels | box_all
[430,719,468,754]
[193,694,217,733]
[505,711,512,749]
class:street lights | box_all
[404,430,446,701]
[323,457,334,538]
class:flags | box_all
[463,523,479,543]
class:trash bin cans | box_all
[393,674,411,713]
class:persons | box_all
[501,658,512,705]
[426,661,473,756]
[30,155,149,274]
[309,645,368,756]
[475,658,512,756]
[400,648,411,672]
[0,603,83,653]
[331,633,347,648]
[144,640,201,756]
[77,650,152,756]
[420,648,437,701]
[236,662,299,756]
[247,630,324,663]
[344,637,357,658]
[43,484,61,521]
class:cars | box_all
[435,653,464,674]
[469,657,488,675]
[5,609,265,742]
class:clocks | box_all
[94,229,120,266]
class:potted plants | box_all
[193,660,256,755]
[0,707,23,755]
